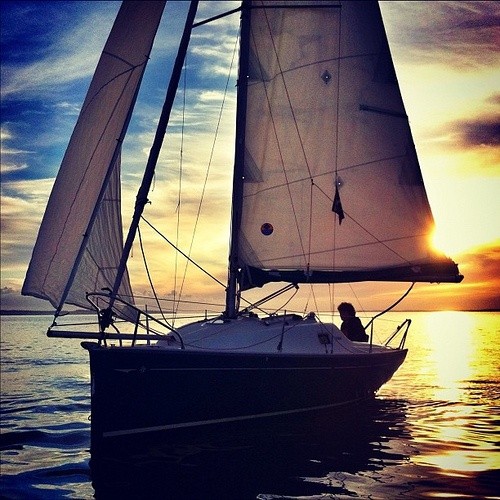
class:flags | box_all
[332,185,346,224]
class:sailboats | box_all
[18,1,465,453]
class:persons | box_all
[337,302,369,342]
